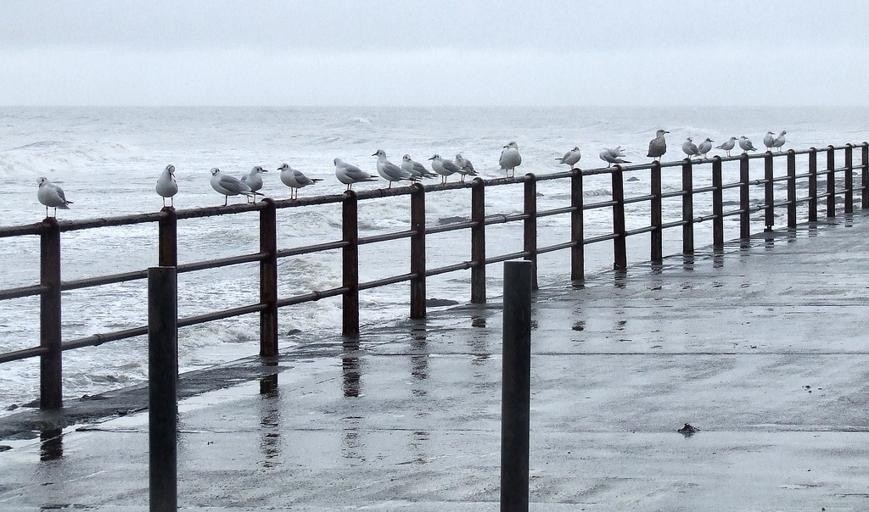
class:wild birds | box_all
[763,129,786,154]
[599,145,632,168]
[154,163,179,211]
[333,156,379,193]
[715,135,758,159]
[498,141,522,181]
[427,151,479,186]
[35,175,72,221]
[646,129,670,163]
[276,163,325,201]
[682,136,715,161]
[209,165,269,206]
[555,146,581,171]
[372,149,438,191]
